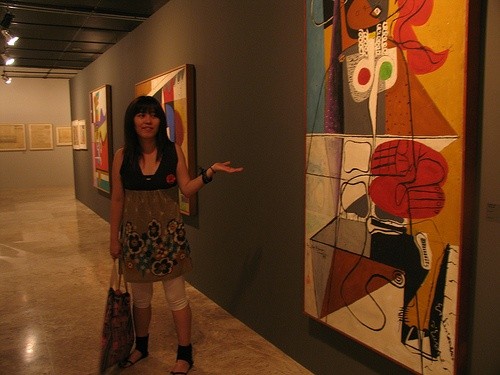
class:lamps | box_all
[1,29,19,46]
[3,75,11,84]
[1,53,15,65]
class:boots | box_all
[121,334,150,367]
[168,343,193,375]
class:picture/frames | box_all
[0,123,26,151]
[28,123,53,150]
[88,84,114,196]
[55,119,88,151]
[134,64,197,217]
[301,0,483,375]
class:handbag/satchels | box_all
[100,254,135,364]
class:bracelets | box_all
[201,167,216,184]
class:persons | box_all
[109,96,245,375]
